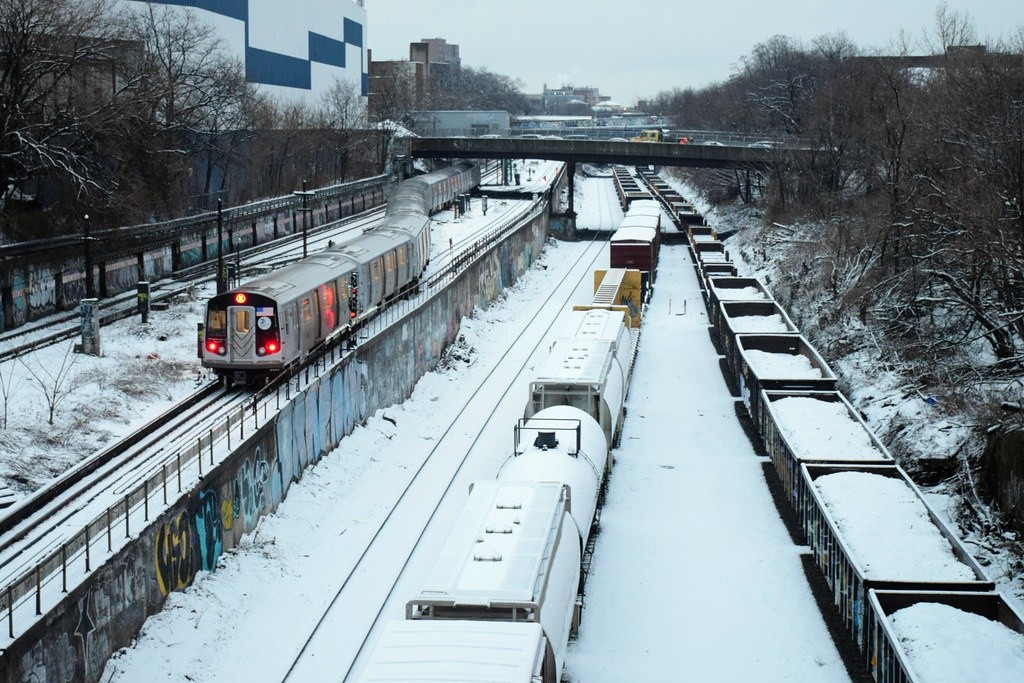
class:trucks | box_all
[630,130,693,144]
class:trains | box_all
[200,160,481,389]
[358,165,662,683]
[635,165,1024,683]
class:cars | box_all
[479,135,628,141]
[702,141,783,147]
